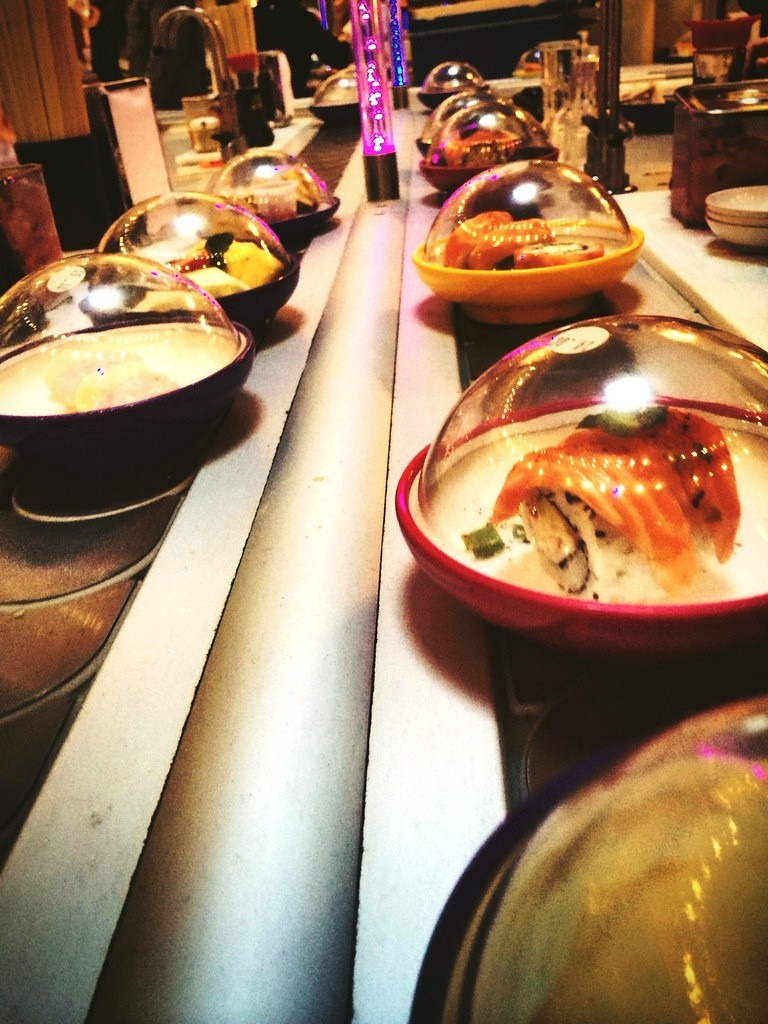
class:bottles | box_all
[182,67,275,152]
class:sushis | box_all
[162,232,283,298]
[443,209,603,271]
[488,405,742,605]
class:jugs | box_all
[538,39,598,174]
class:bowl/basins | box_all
[267,191,345,245]
[394,392,768,650]
[1,321,259,460]
[414,220,645,326]
[705,186,768,253]
[408,681,768,1024]
[308,58,563,193]
[79,257,307,340]
